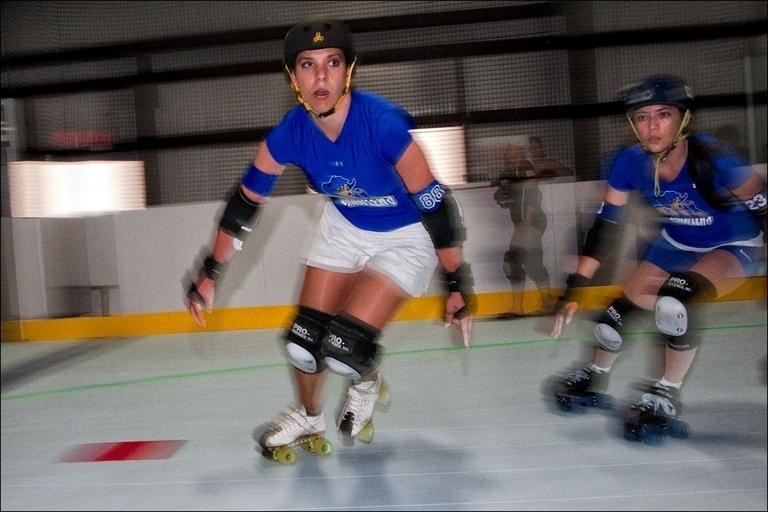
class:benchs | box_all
[50,282,122,318]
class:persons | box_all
[182,16,479,449]
[548,73,767,426]
[488,137,557,318]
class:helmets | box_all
[623,73,697,116]
[283,19,355,78]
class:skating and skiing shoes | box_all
[336,369,391,448]
[621,381,692,448]
[257,402,333,465]
[553,361,613,412]
